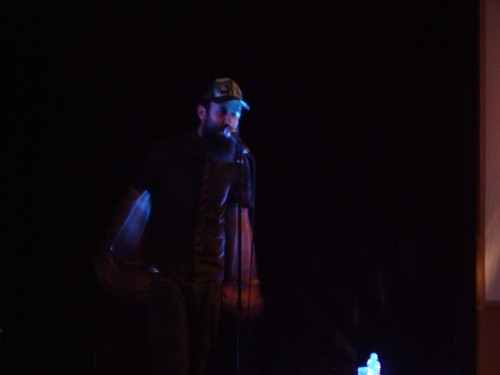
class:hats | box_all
[207,78,250,110]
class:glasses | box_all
[208,108,241,119]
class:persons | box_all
[93,77,262,375]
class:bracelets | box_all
[99,241,113,251]
[232,278,260,289]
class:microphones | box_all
[220,122,254,163]
